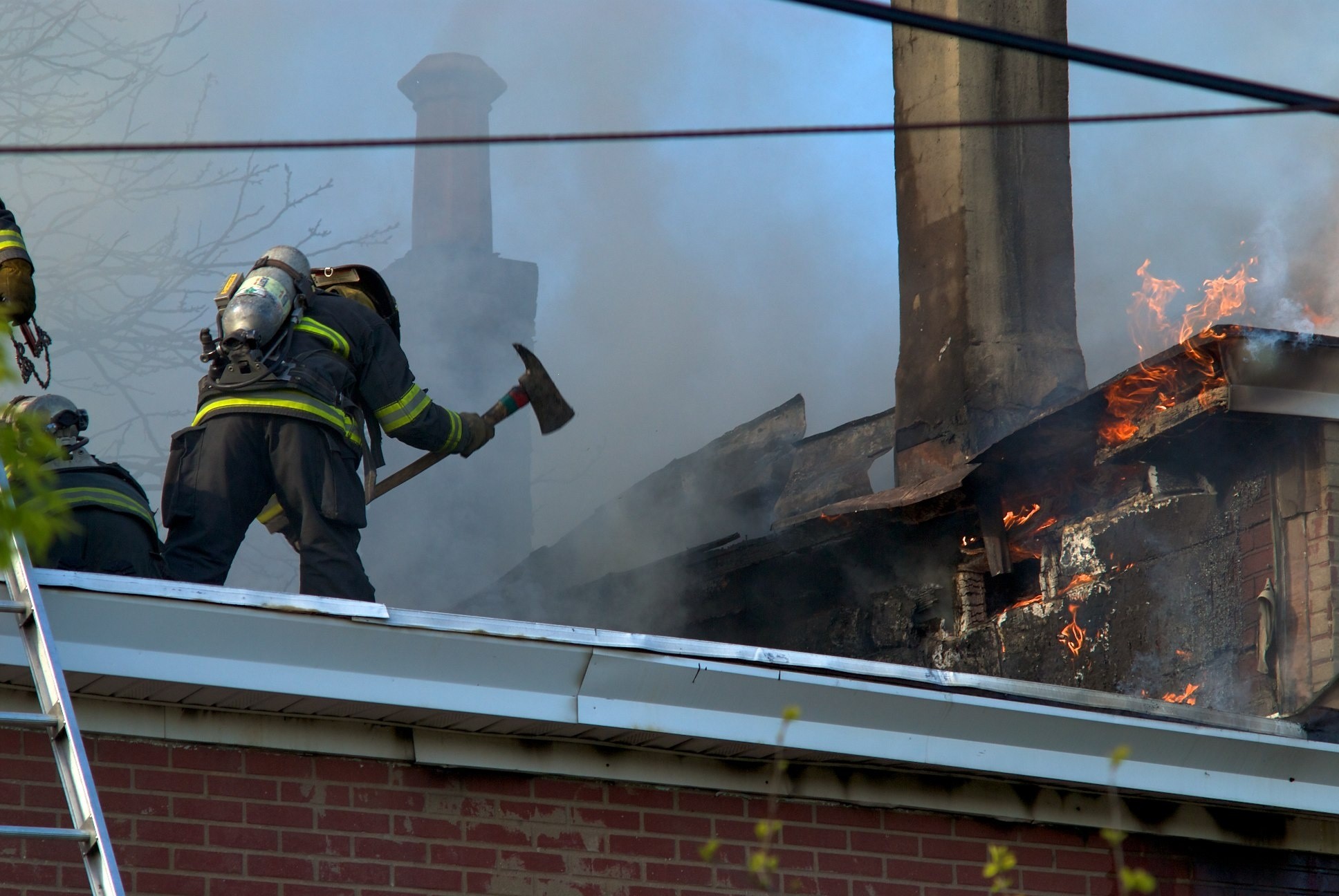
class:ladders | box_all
[0,460,137,896]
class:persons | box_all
[0,198,36,327]
[158,264,495,603]
[0,395,165,579]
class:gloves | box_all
[462,412,495,458]
[282,516,299,550]
[0,259,36,326]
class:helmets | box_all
[10,393,80,456]
[312,265,400,337]
